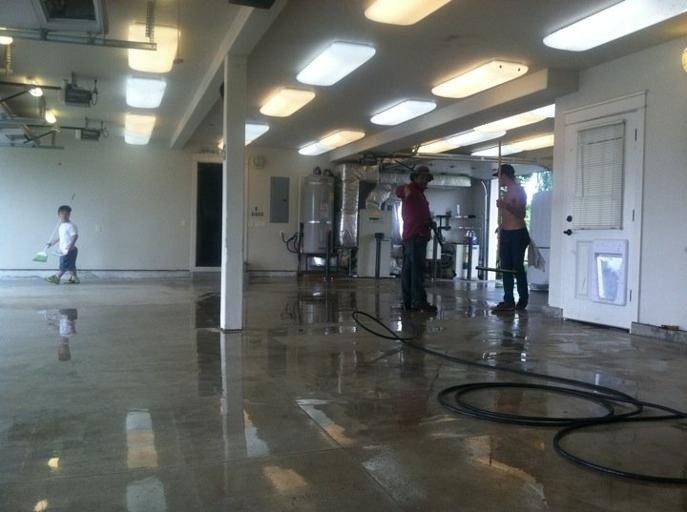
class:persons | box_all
[490,164,531,312]
[388,315,441,441]
[47,308,79,361]
[395,166,438,314]
[47,205,81,284]
[496,312,529,416]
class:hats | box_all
[409,165,434,182]
[492,164,514,177]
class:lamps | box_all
[543,0,686,53]
[126,22,181,73]
[432,56,530,99]
[126,75,167,109]
[261,87,316,119]
[365,1,449,27]
[295,39,378,86]
[295,128,365,156]
[123,113,156,146]
[215,120,272,151]
[368,98,438,126]
[409,102,555,159]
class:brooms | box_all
[31,192,76,262]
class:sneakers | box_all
[515,298,528,310]
[44,275,60,285]
[490,302,515,311]
[63,275,81,284]
[410,300,437,312]
